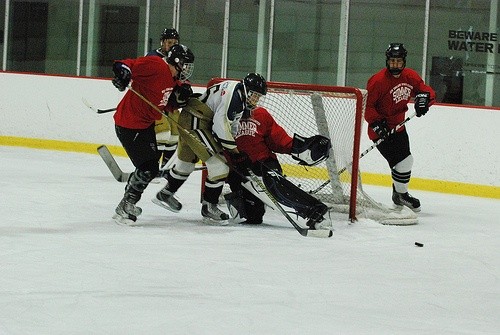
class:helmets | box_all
[160,28,180,44]
[239,72,268,110]
[385,43,408,75]
[165,44,195,83]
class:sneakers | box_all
[113,197,142,226]
[152,188,182,213]
[392,183,422,212]
[201,200,230,226]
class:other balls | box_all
[415,242,423,247]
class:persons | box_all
[365,43,436,212]
[152,71,334,232]
[111,44,195,226]
[144,28,180,184]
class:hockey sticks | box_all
[96,145,208,183]
[126,84,263,183]
[297,111,417,195]
[247,167,333,238]
[81,94,118,115]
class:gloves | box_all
[169,84,193,109]
[231,150,253,173]
[414,90,431,117]
[291,133,332,166]
[370,118,393,144]
[111,62,132,91]
